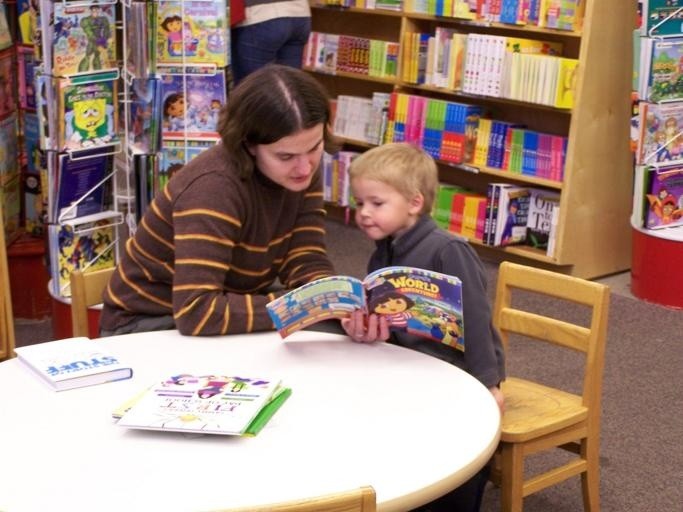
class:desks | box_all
[0,328,507,512]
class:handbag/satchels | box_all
[229,0,245,26]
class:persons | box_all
[369,289,415,335]
[226,0,312,85]
[345,141,511,512]
[97,61,391,345]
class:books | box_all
[301,0,585,255]
[629,1,682,230]
[121,369,282,437]
[1,0,229,296]
[12,333,134,394]
[265,264,467,358]
[113,376,292,437]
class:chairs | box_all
[489,260,611,512]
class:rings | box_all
[354,333,364,339]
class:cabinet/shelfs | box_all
[302,2,640,282]
